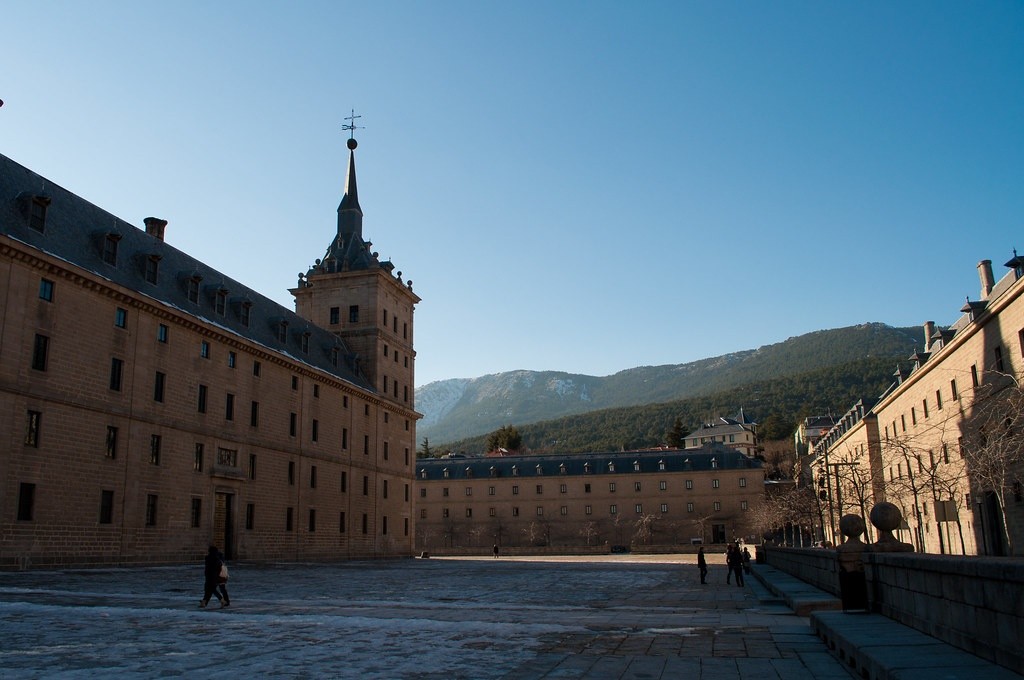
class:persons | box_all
[217,552,230,607]
[493,544,499,559]
[727,545,736,584]
[198,546,228,609]
[730,547,744,587]
[741,547,751,575]
[697,547,708,584]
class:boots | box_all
[197,599,207,608]
[220,597,230,609]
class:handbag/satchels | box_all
[219,561,228,581]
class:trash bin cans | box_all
[841,570,868,609]
[755,546,764,563]
